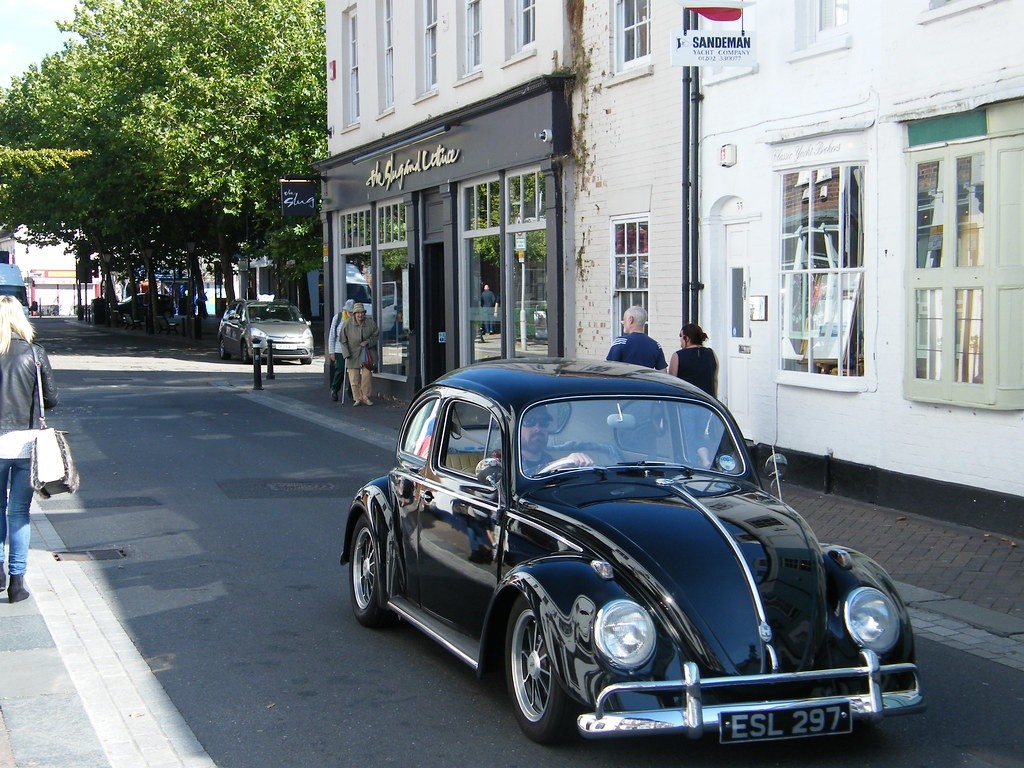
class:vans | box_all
[0,263,29,322]
[345,264,398,338]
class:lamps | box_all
[351,125,451,166]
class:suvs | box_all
[120,292,177,320]
[217,299,315,365]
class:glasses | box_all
[522,419,549,429]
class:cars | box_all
[339,357,927,745]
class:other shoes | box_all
[330,388,338,401]
[353,402,361,406]
[363,399,373,406]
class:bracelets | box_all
[495,312,498,313]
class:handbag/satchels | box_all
[360,347,374,370]
[30,428,80,498]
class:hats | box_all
[345,299,355,313]
[352,303,365,314]
[522,405,554,421]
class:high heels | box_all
[0,562,7,592]
[7,575,30,603]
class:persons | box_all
[248,288,254,299]
[668,324,719,467]
[520,405,593,476]
[339,303,379,407]
[481,285,498,333]
[328,299,354,401]
[199,290,208,319]
[606,306,666,453]
[0,294,59,604]
[250,308,257,317]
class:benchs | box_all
[121,313,144,331]
[446,451,615,470]
[154,316,180,336]
